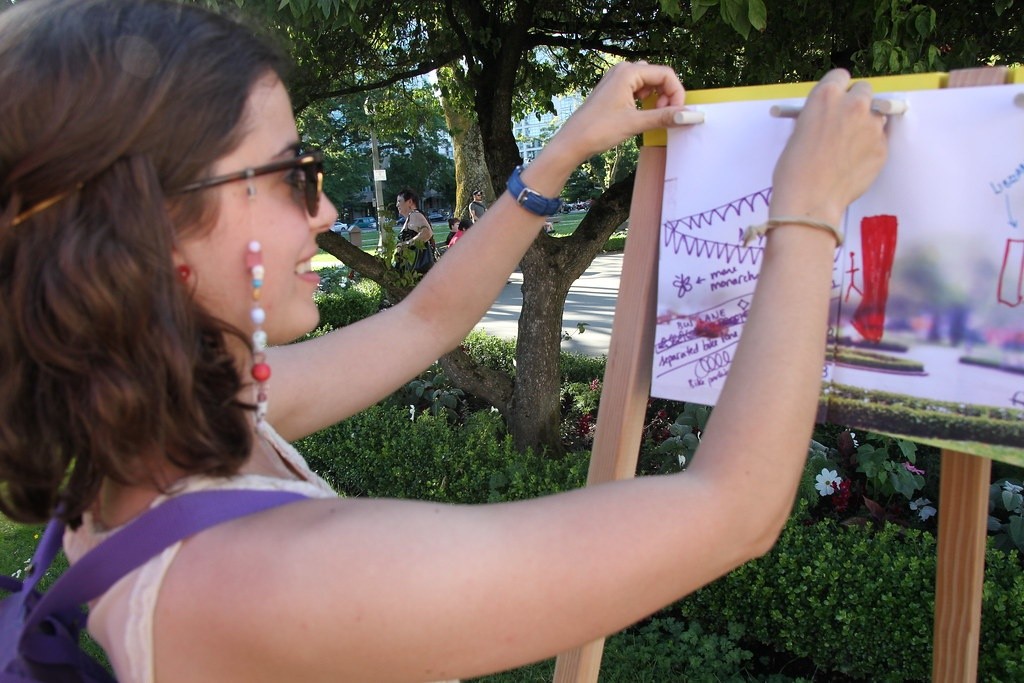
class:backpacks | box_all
[0,456,309,683]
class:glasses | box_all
[179,139,324,217]
[478,195,482,196]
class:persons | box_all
[396,189,434,280]
[469,190,487,223]
[588,195,596,206]
[545,223,555,233]
[1,0,887,683]
[446,218,472,247]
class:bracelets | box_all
[507,167,560,214]
[746,218,842,248]
[397,243,404,247]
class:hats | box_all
[473,190,483,195]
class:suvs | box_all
[351,217,376,228]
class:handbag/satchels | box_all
[393,210,434,272]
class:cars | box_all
[397,216,406,225]
[333,222,348,232]
[426,213,448,222]
